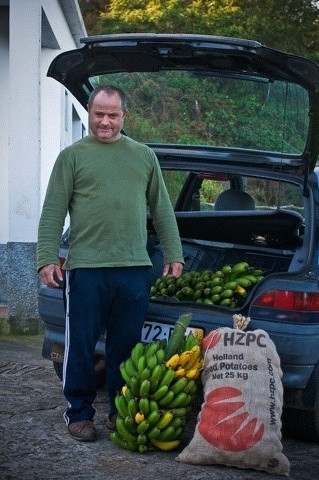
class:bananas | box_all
[111,333,203,452]
[149,262,264,308]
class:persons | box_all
[36,86,186,442]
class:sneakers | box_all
[106,412,117,428]
[68,420,96,440]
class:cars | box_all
[38,32,316,438]
